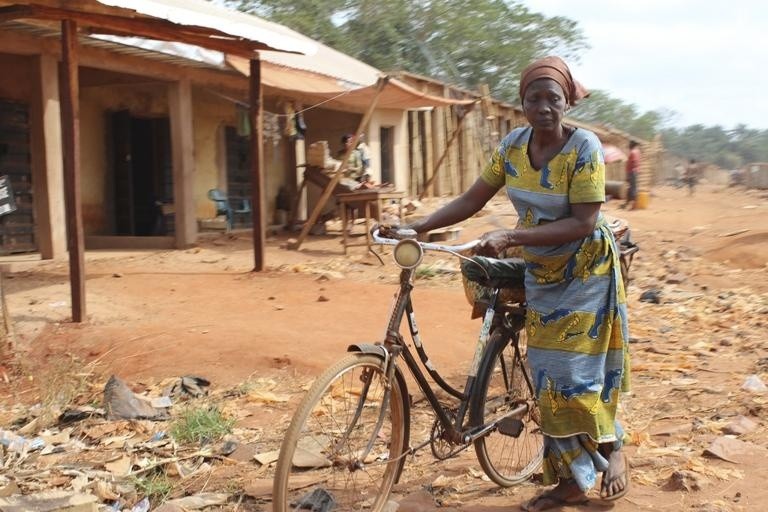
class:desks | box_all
[337,191,405,254]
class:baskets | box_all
[457,241,638,309]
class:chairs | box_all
[207,189,252,228]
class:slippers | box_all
[520,491,589,512]
[601,455,628,501]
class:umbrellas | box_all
[600,144,628,166]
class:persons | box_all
[333,134,364,232]
[684,159,698,195]
[352,131,371,185]
[619,140,642,210]
[367,54,636,512]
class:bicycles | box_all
[270,230,640,511]
